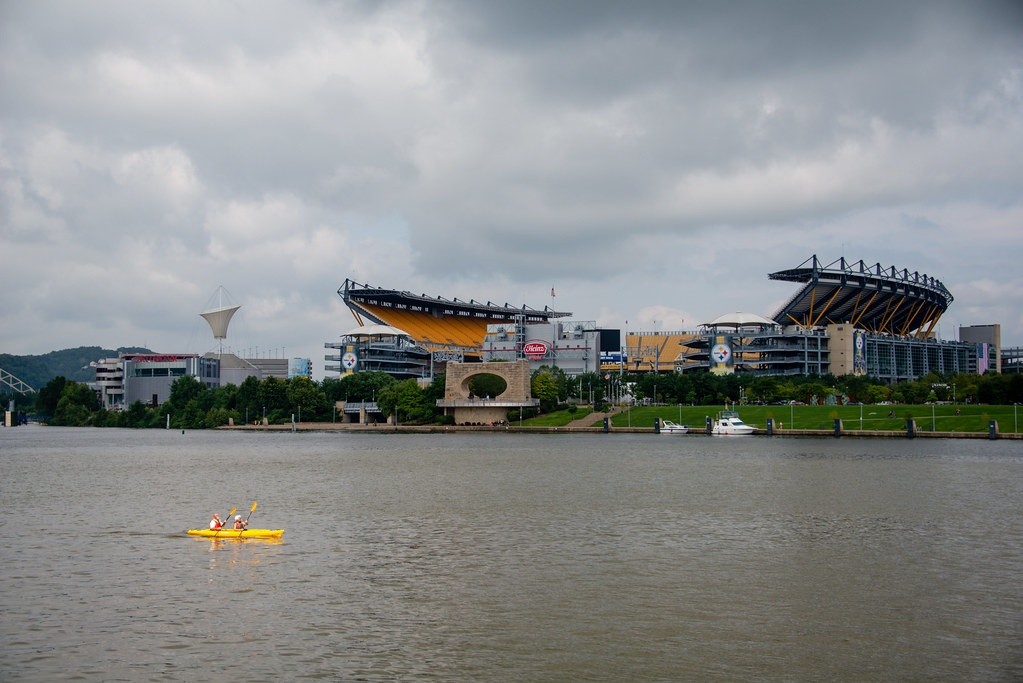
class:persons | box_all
[779,420,782,429]
[209,514,226,531]
[956,407,960,416]
[233,515,248,531]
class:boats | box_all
[711,401,757,435]
[660,421,689,434]
[187,528,284,539]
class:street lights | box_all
[333,406,336,424]
[679,403,682,425]
[246,408,248,424]
[953,383,955,405]
[263,407,265,419]
[931,403,935,432]
[1014,403,1017,433]
[860,403,863,431]
[298,406,300,424]
[627,404,631,428]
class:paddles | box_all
[214,506,237,537]
[239,501,257,538]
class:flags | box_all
[551,288,555,296]
[605,372,621,385]
